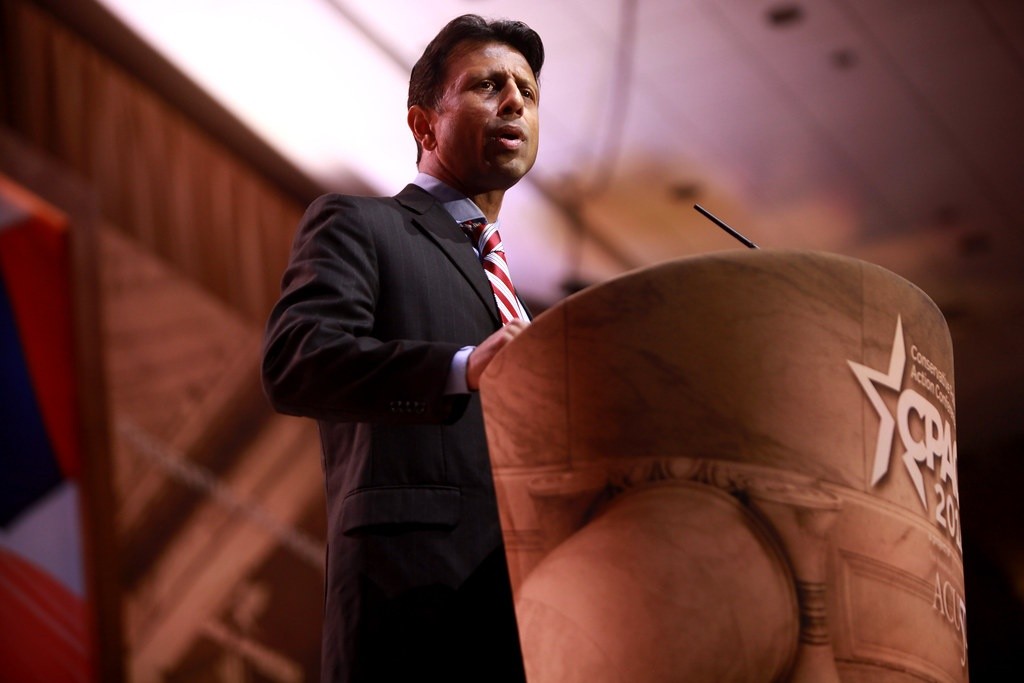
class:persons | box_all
[261,12,545,683]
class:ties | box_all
[460,220,525,327]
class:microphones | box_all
[692,203,759,248]
